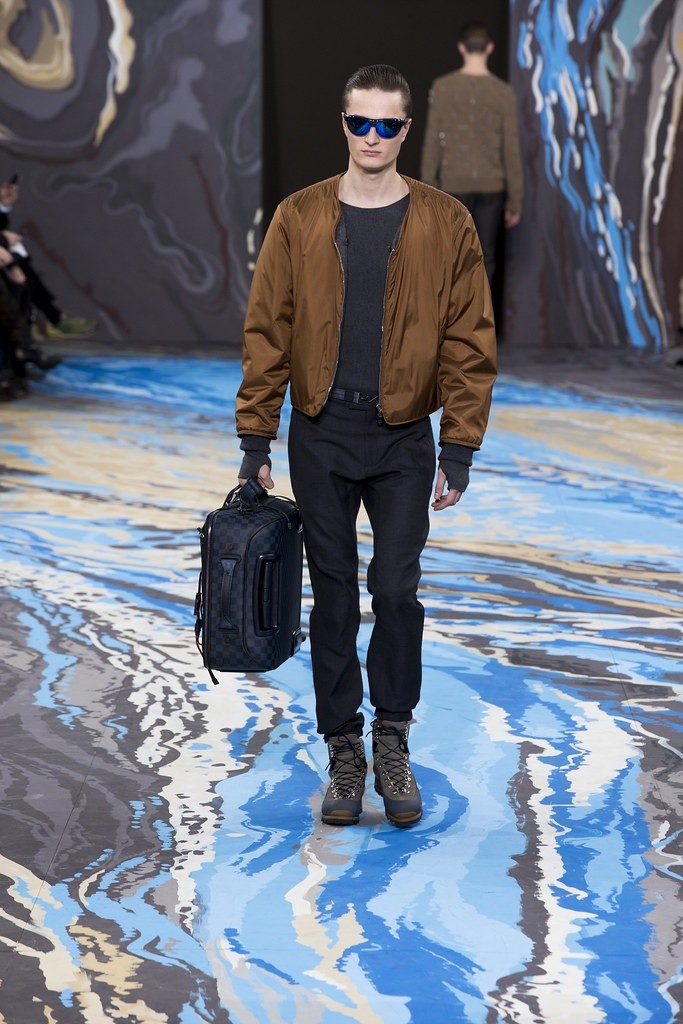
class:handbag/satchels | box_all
[194,479,304,685]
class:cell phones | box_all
[8,172,23,195]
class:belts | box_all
[330,388,362,403]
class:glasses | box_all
[343,114,408,139]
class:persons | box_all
[233,65,499,830]
[0,181,86,403]
[418,28,523,291]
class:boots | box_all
[320,733,367,826]
[365,719,422,827]
